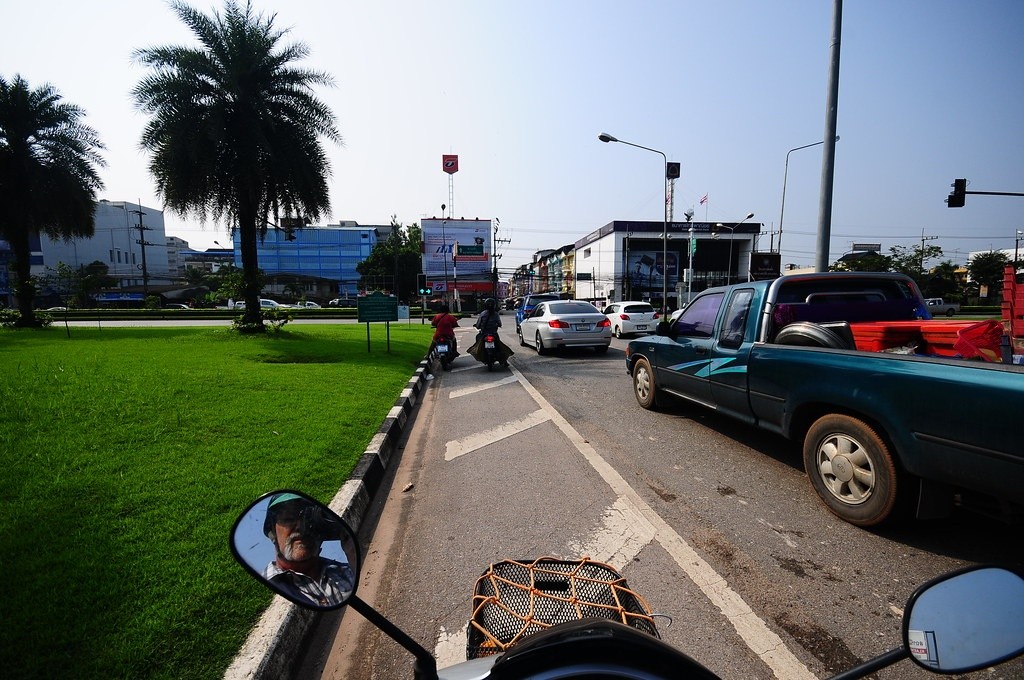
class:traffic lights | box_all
[944,179,966,209]
[417,274,433,297]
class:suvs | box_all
[516,295,560,332]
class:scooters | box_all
[428,316,462,372]
[473,323,501,371]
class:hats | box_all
[262,495,321,538]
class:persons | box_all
[424,305,460,358]
[475,298,508,368]
[263,493,357,608]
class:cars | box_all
[234,299,320,309]
[518,300,611,356]
[601,300,659,339]
[329,299,357,307]
[166,303,188,309]
[501,297,524,309]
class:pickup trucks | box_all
[623,271,1024,525]
[924,298,960,317]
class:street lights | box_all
[442,204,448,314]
[717,213,755,286]
[214,241,231,273]
[597,133,668,322]
[779,135,841,253]
[684,209,693,304]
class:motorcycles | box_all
[229,489,1024,680]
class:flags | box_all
[700,195,707,205]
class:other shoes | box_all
[501,364,509,367]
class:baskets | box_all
[465,556,662,662]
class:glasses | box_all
[274,509,318,524]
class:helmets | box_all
[484,299,496,310]
